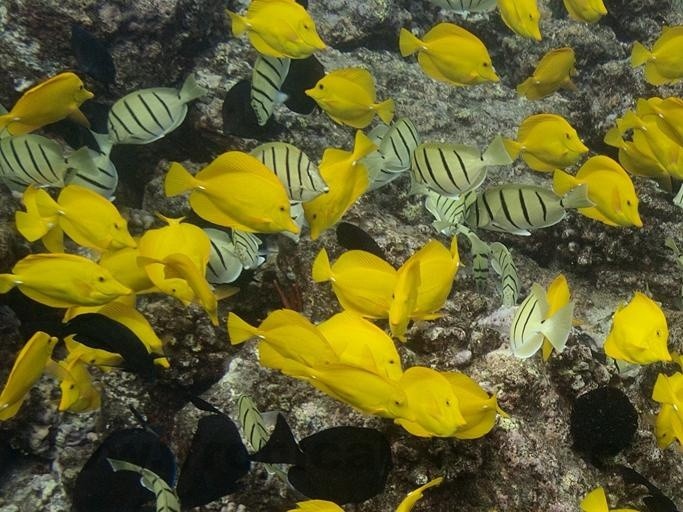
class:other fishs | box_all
[0,0,683,512]
